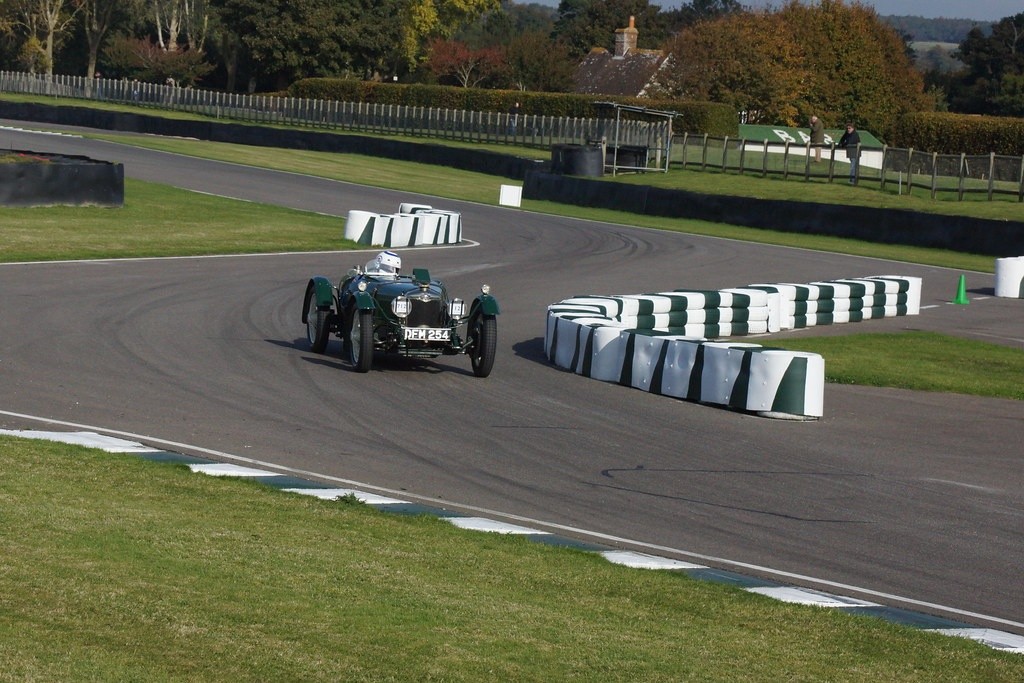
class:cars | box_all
[301,266,500,378]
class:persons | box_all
[838,124,862,186]
[366,250,400,283]
[810,115,824,161]
[94,71,103,100]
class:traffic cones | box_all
[951,274,970,305]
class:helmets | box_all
[375,251,402,277]
